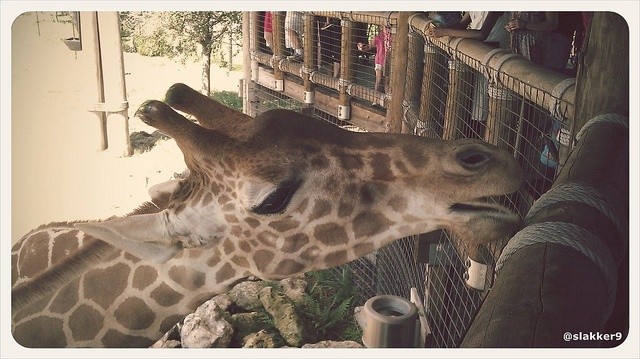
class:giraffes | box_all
[10,82,526,347]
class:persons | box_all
[422,11,592,175]
[262,11,273,57]
[283,11,304,62]
[357,26,389,106]
[330,18,341,78]
